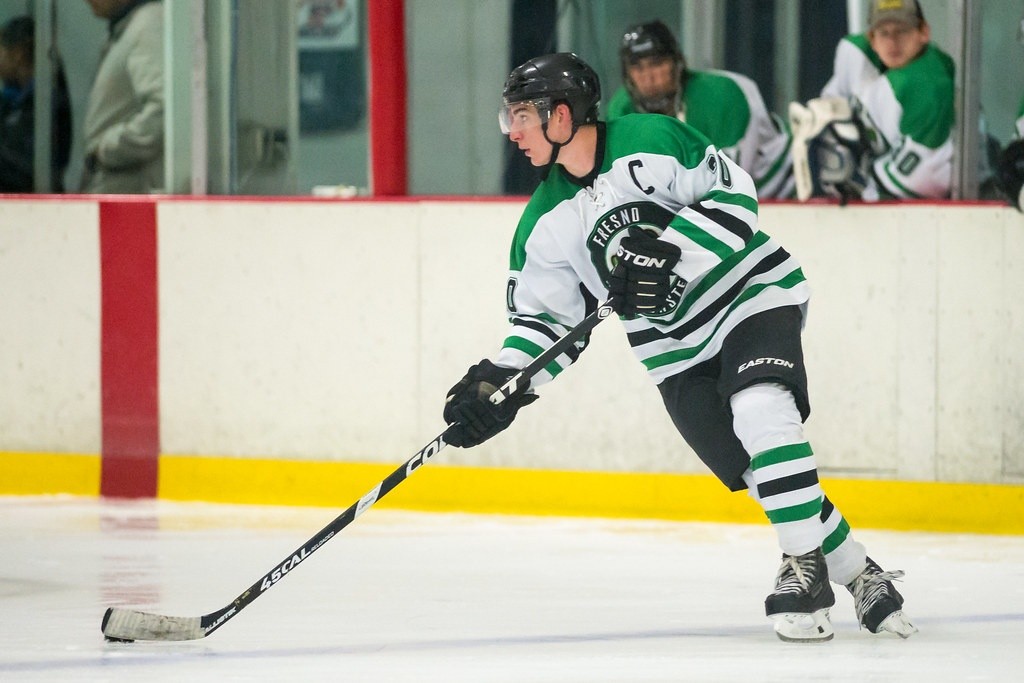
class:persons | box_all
[443,51,921,641]
[607,0,1024,213]
[0,0,167,194]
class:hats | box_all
[869,0,925,29]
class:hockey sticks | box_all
[98,293,622,647]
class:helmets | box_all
[501,51,601,125]
[620,19,680,116]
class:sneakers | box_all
[843,556,917,638]
[765,546,836,641]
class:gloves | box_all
[605,226,681,320]
[442,358,539,448]
